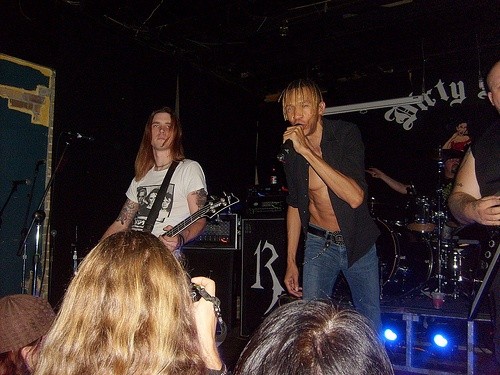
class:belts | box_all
[307,225,344,245]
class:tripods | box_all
[403,172,471,300]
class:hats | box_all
[0,294,56,354]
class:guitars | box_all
[162,192,240,237]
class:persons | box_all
[101,107,208,260]
[0,293,57,375]
[234,299,396,375]
[282,76,383,340]
[27,230,229,375]
[365,149,463,200]
[448,61,500,227]
[137,188,172,222]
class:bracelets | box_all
[179,234,184,246]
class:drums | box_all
[406,195,440,232]
[374,217,433,297]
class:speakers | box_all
[182,248,235,336]
[239,216,300,337]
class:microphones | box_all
[277,123,303,162]
[66,132,95,142]
[12,179,32,185]
